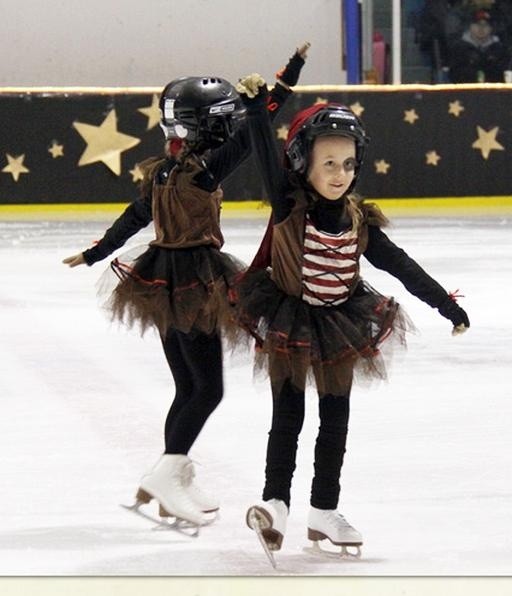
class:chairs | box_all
[409,3,454,82]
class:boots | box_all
[138,455,217,526]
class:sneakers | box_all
[247,499,287,551]
[306,504,363,547]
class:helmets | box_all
[286,103,366,178]
[160,78,245,147]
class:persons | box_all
[232,71,473,550]
[62,39,317,527]
[417,0,512,84]
